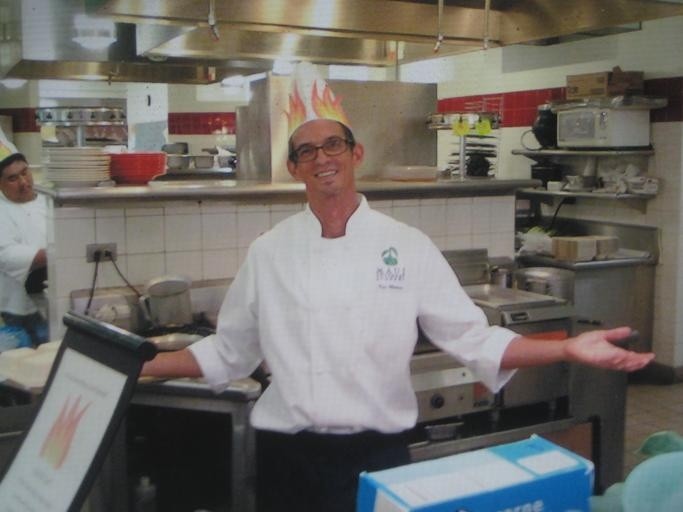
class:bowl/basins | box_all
[564,176,578,186]
[422,418,464,439]
[167,153,235,169]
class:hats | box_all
[287,61,355,142]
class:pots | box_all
[516,266,575,302]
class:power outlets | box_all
[86,242,117,263]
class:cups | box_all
[426,112,498,130]
[137,273,194,329]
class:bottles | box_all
[34,110,126,120]
[533,104,558,149]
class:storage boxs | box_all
[566,72,645,101]
[354,434,594,512]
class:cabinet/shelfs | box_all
[512,150,657,214]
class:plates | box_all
[42,145,167,189]
[563,186,593,192]
[550,234,620,264]
[403,440,429,449]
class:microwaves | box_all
[551,103,654,151]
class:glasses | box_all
[288,135,353,163]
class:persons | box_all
[137,117,658,512]
[0,150,56,408]
